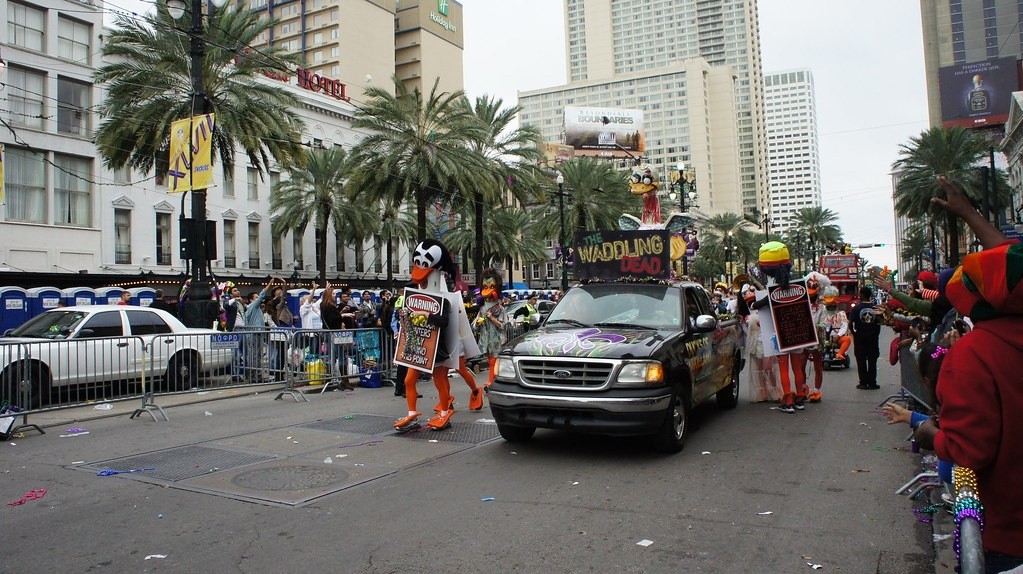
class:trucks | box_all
[819,254,859,317]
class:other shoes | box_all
[401,392,423,398]
[856,383,867,389]
[867,382,880,390]
[394,389,402,396]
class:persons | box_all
[510,294,516,301]
[825,242,852,254]
[728,241,829,412]
[847,287,882,388]
[654,297,695,326]
[391,279,482,431]
[118,292,130,305]
[150,289,168,309]
[550,291,563,302]
[223,277,404,390]
[872,175,1023,574]
[57,301,67,308]
[831,312,851,360]
[471,294,509,393]
[539,292,544,298]
[712,292,727,315]
[511,295,543,332]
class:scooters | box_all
[819,325,850,371]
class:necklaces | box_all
[953,467,983,561]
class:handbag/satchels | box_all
[286,344,304,368]
[332,331,353,344]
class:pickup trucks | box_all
[487,281,746,455]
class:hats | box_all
[271,286,282,298]
[525,293,535,300]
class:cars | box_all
[505,300,557,334]
[0,305,235,412]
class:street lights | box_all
[668,161,696,274]
[546,174,575,293]
[165,0,219,329]
[722,231,738,284]
[758,207,775,243]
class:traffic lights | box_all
[874,244,885,247]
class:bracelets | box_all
[885,286,891,292]
